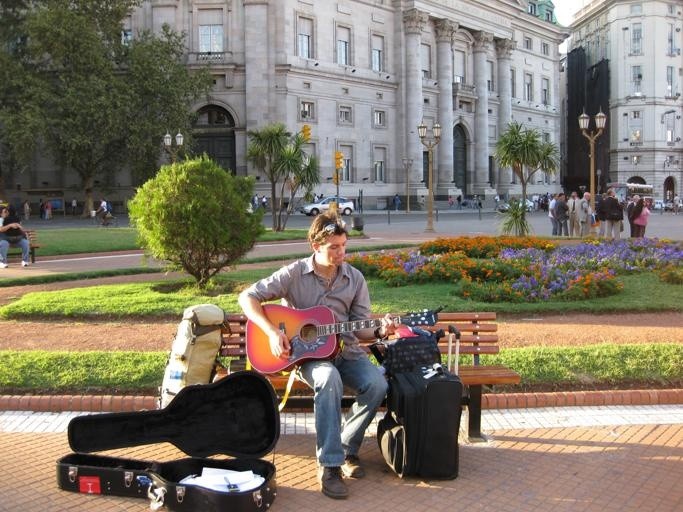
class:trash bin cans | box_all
[352,214,364,231]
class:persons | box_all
[536,189,651,238]
[313,193,323,203]
[494,194,501,210]
[0,207,30,268]
[236,210,400,499]
[0,205,24,244]
[394,194,401,211]
[252,193,267,209]
[96,197,108,226]
[418,195,426,211]
[0,197,52,222]
[446,191,483,210]
[70,196,78,215]
[674,194,679,214]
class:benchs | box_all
[7,230,42,264]
[211,307,522,446]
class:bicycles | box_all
[89,209,117,228]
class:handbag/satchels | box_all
[591,213,600,228]
[620,220,624,232]
[557,214,569,224]
[381,325,444,385]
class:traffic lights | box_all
[335,150,343,169]
[303,124,311,143]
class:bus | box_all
[605,181,654,210]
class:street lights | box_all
[415,120,442,234]
[577,104,608,216]
[163,128,184,163]
[401,156,413,213]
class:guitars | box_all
[246,304,449,376]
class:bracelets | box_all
[373,327,389,341]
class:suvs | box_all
[296,194,355,216]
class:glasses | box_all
[312,223,345,243]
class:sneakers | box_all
[318,464,349,499]
[340,454,365,479]
[0,260,29,269]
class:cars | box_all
[497,196,534,213]
[591,192,683,211]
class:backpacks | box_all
[1,214,24,242]
[158,304,231,410]
[102,201,112,212]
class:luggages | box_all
[376,324,463,481]
